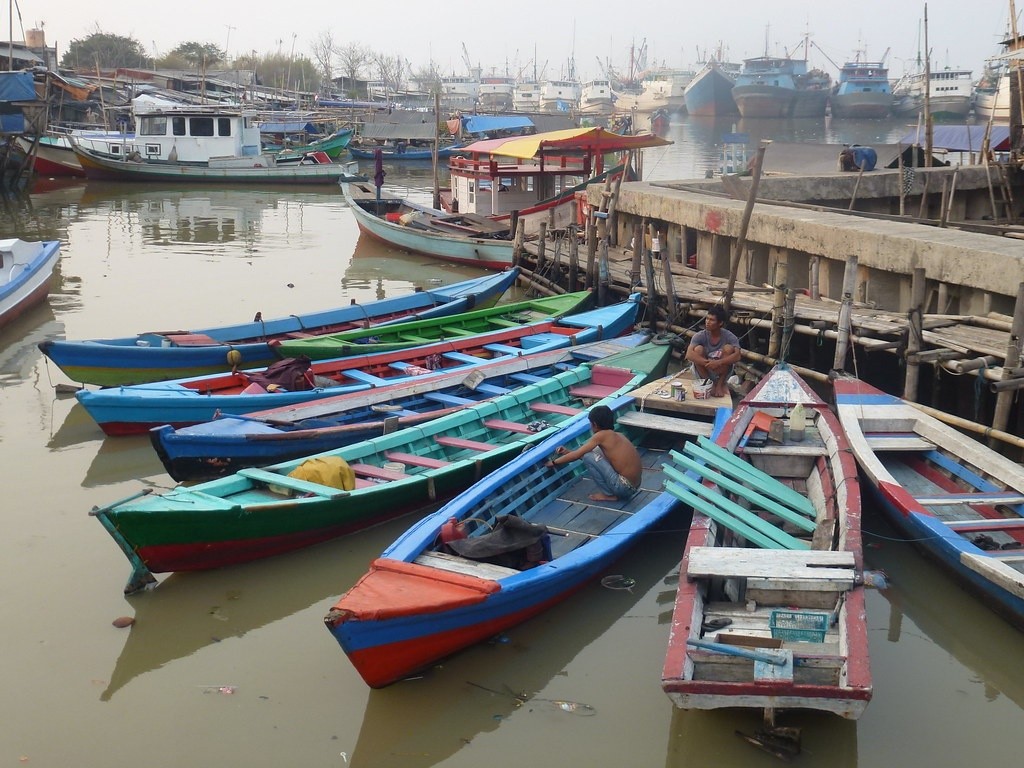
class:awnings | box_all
[460,126,674,162]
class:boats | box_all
[16,42,541,184]
[627,37,695,112]
[0,233,62,329]
[731,19,797,119]
[322,358,735,688]
[971,0,1024,125]
[611,86,669,115]
[335,173,509,270]
[37,265,523,387]
[87,331,681,596]
[146,328,655,488]
[578,80,616,117]
[683,39,735,117]
[434,125,676,239]
[893,1,975,118]
[827,26,893,118]
[788,23,832,117]
[538,56,581,116]
[76,291,646,439]
[659,363,875,726]
[831,368,1023,641]
[718,123,753,175]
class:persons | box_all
[685,306,742,398]
[489,128,513,139]
[543,405,643,502]
[520,126,537,136]
[396,139,406,154]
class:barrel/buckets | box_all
[384,462,405,474]
[441,517,467,554]
[790,404,806,441]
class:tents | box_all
[466,116,537,133]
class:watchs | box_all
[552,459,556,465]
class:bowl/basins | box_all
[691,379,713,399]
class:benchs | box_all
[178,297,1024,587]
